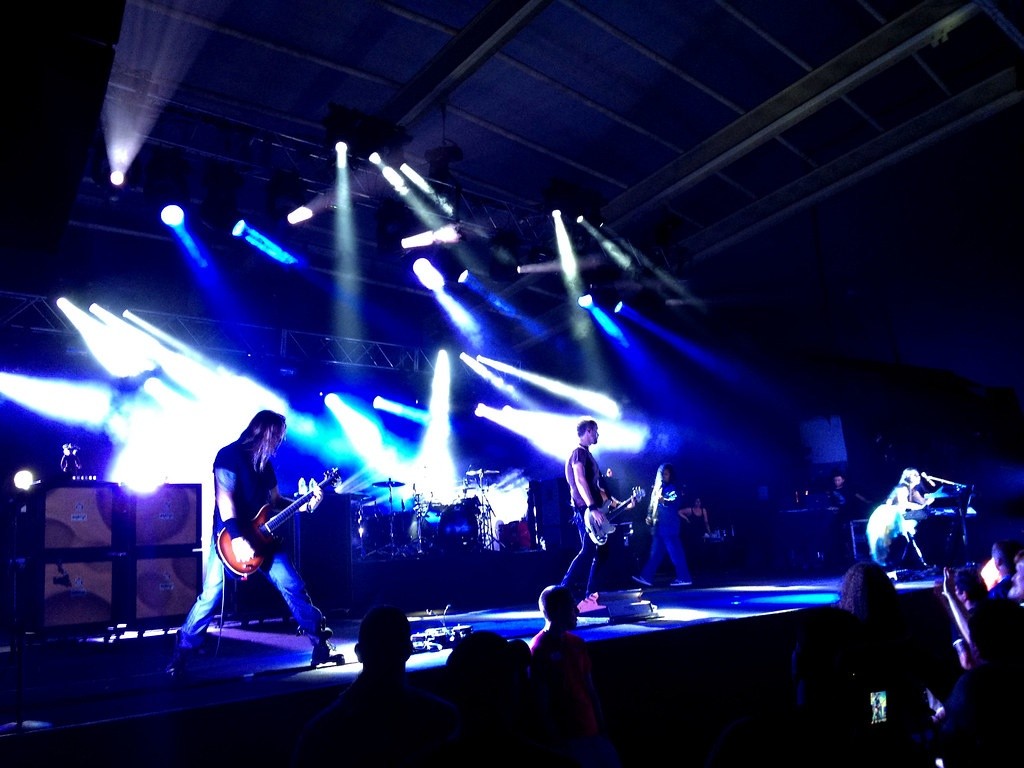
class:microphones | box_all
[921,471,936,487]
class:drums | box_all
[420,504,479,549]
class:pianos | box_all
[902,485,978,575]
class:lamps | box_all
[199,197,248,240]
[446,261,469,284]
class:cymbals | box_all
[467,469,500,475]
[372,480,405,487]
[458,486,476,490]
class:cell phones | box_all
[870,690,887,724]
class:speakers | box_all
[125,482,205,550]
[25,554,122,636]
[25,481,123,554]
[123,551,202,631]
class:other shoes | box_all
[670,578,693,587]
[632,575,653,586]
[167,660,190,676]
[308,626,334,644]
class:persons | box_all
[176,411,332,649]
[678,498,711,577]
[703,505,1024,768]
[560,421,636,605]
[632,463,692,586]
[293,584,620,768]
[827,476,872,511]
[886,468,935,566]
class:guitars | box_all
[584,485,646,546]
[648,490,677,535]
[215,466,342,579]
